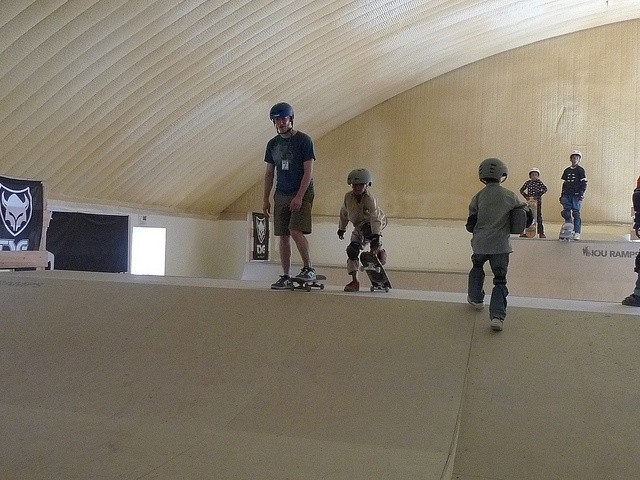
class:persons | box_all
[559,150,588,240]
[337,168,388,292]
[622,172,640,307]
[519,167,547,239]
[262,101,317,290]
[465,158,526,333]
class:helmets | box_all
[347,168,372,187]
[270,102,294,121]
[479,158,508,180]
[569,150,582,159]
[528,167,540,176]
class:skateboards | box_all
[359,252,393,293]
[524,194,538,238]
[288,275,327,292]
[558,223,575,242]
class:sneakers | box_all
[378,249,387,265]
[539,234,546,238]
[344,281,359,292]
[271,274,293,290]
[622,294,640,306]
[296,266,317,282]
[466,295,485,310]
[520,234,527,237]
[573,233,581,240]
[490,318,504,331]
